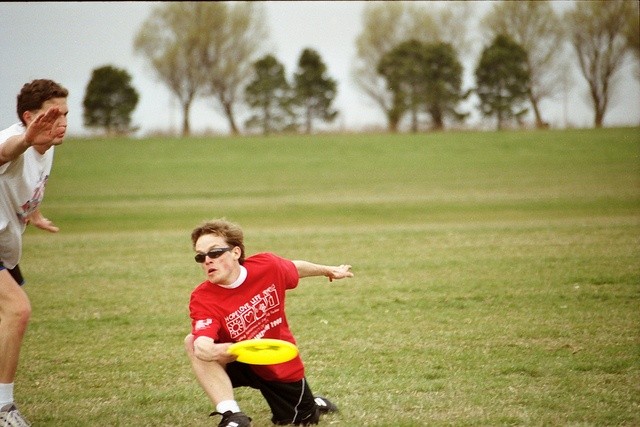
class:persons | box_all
[183,218,356,427]
[0,80,70,427]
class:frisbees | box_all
[227,339,299,366]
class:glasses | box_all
[195,248,232,262]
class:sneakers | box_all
[0,403,30,427]
[210,410,252,427]
[317,396,338,413]
